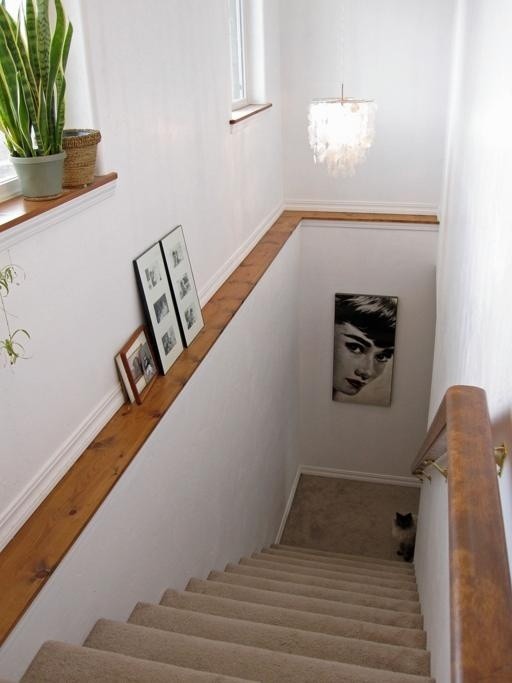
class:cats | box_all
[391,511,418,563]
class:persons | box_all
[332,292,397,403]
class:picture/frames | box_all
[112,324,157,408]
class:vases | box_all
[58,126,103,187]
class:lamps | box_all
[303,86,380,177]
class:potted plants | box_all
[0,0,75,202]
